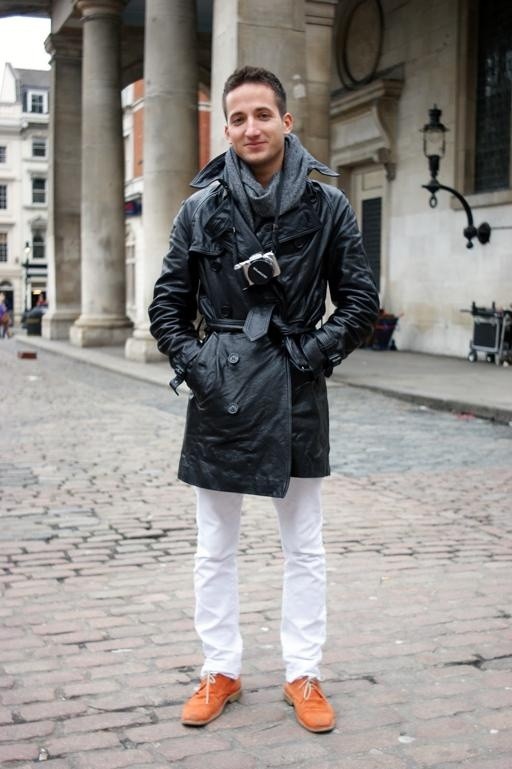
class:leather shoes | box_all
[283,676,336,732]
[182,674,243,725]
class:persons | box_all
[147,64,384,731]
[0,290,15,339]
[36,291,48,307]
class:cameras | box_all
[233,251,282,293]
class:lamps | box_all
[418,102,491,250]
